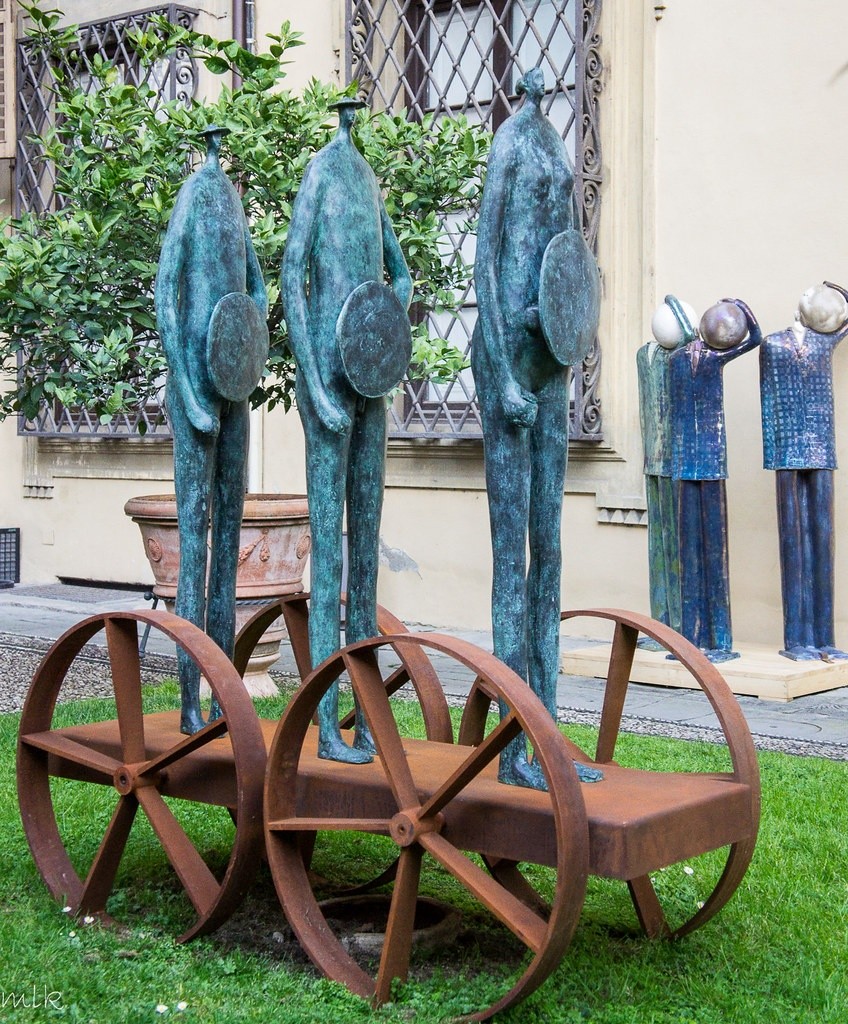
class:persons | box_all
[154,124,270,740]
[279,95,414,764]
[636,280,848,661]
[469,67,601,793]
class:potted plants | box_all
[0,0,493,699]
